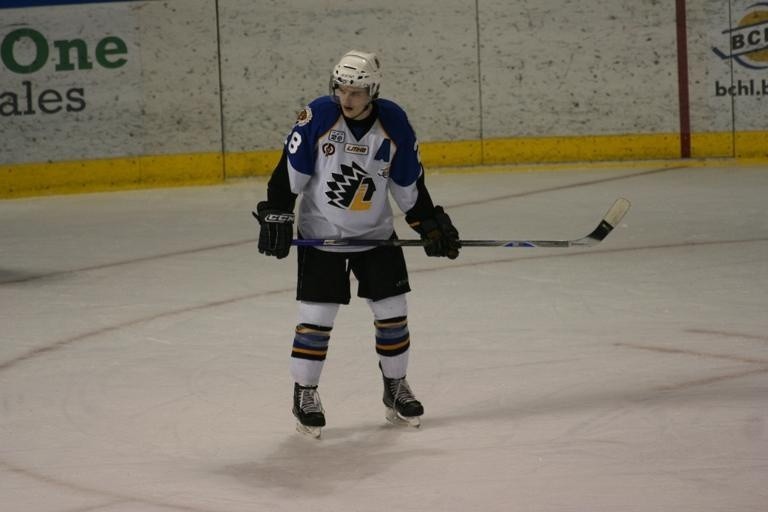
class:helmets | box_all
[328,48,381,97]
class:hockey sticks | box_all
[291,197,631,248]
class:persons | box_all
[253,50,463,427]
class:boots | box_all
[377,361,423,419]
[291,381,325,428]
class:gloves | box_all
[404,202,459,259]
[256,200,293,258]
